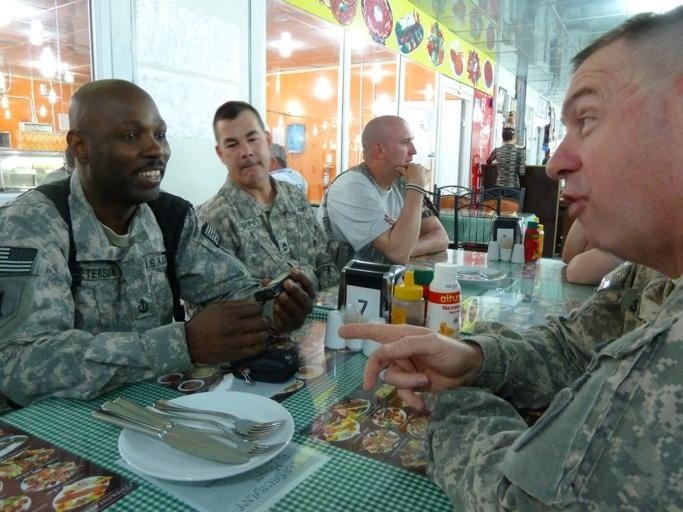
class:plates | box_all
[154,365,218,390]
[457,265,508,284]
[514,306,534,315]
[118,391,295,483]
[324,397,430,470]
[276,365,327,396]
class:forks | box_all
[146,399,288,456]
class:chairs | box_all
[432,184,525,249]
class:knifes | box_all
[92,398,251,465]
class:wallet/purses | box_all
[231,348,300,384]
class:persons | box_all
[315,115,450,263]
[559,216,623,286]
[267,143,307,198]
[485,126,525,202]
[338,0,681,511]
[0,80,314,406]
[36,145,75,188]
[180,100,347,309]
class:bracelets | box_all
[404,183,424,193]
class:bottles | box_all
[391,271,425,328]
[524,218,545,263]
[425,262,461,341]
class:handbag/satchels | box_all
[326,238,353,271]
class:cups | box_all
[363,317,387,357]
[510,244,525,264]
[324,310,346,350]
[486,240,500,261]
[499,247,512,261]
